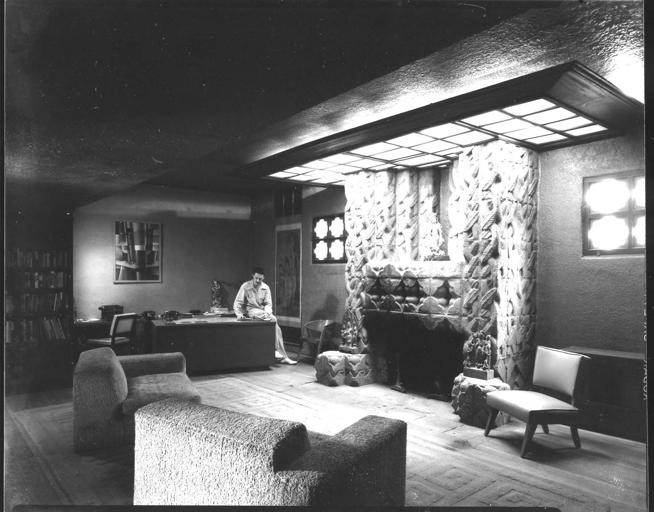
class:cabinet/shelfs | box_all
[4,244,73,395]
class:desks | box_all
[149,317,276,373]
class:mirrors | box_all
[113,218,163,285]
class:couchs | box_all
[131,396,409,509]
[71,349,202,452]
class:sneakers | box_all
[275,350,282,359]
[280,358,298,364]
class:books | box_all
[4,244,72,353]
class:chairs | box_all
[85,310,137,354]
[483,343,595,463]
[295,318,338,366]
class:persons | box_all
[231,264,298,366]
